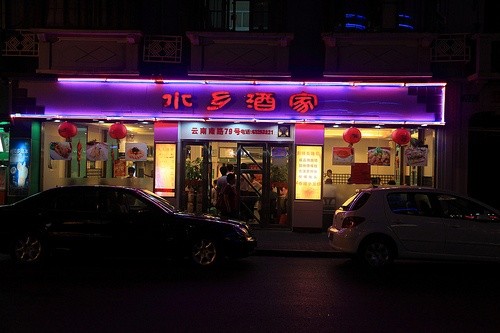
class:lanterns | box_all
[57,121,77,142]
[109,121,127,149]
[391,127,411,148]
[343,126,361,147]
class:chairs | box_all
[419,200,432,216]
[407,200,418,213]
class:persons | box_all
[325,169,332,184]
[211,163,256,219]
[371,177,396,185]
[271,187,277,206]
[125,167,137,178]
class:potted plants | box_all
[270,164,288,188]
[184,165,203,188]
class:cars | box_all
[0,184,258,277]
[327,184,500,277]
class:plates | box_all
[367,149,390,166]
[406,148,428,166]
[50,143,72,161]
[86,143,108,162]
[125,143,147,161]
